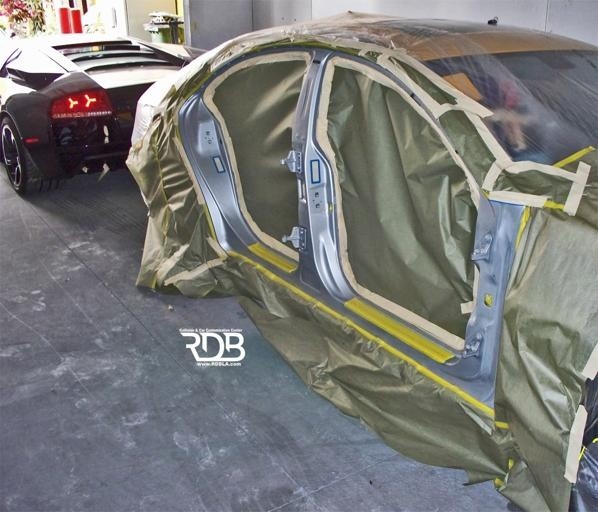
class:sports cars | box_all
[0,35,208,198]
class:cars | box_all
[125,12,598,512]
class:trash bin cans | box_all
[142,11,184,43]
